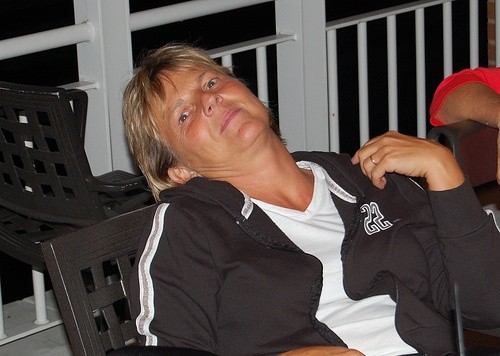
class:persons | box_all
[103,39,500,356]
[427,67,500,184]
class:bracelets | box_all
[370,155,377,166]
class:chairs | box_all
[0,84,164,356]
